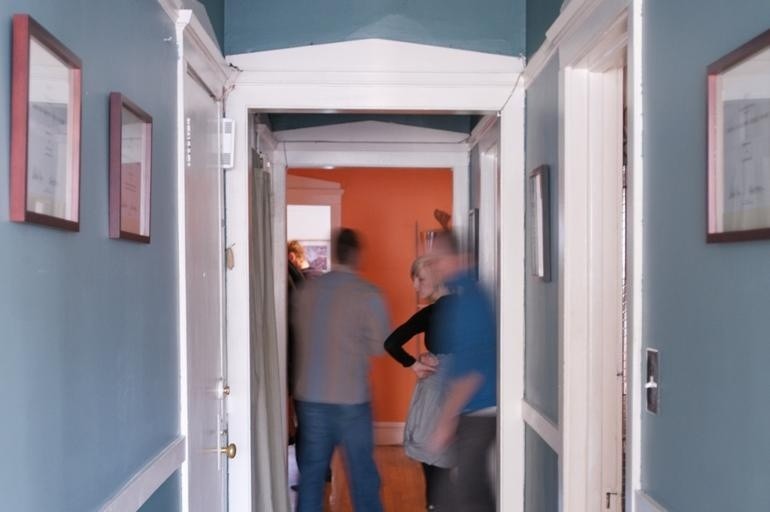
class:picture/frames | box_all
[468,208,479,282]
[529,164,551,283]
[109,91,153,243]
[297,239,330,274]
[706,28,770,244]
[10,14,83,232]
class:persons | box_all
[287,241,332,492]
[288,228,391,512]
[418,233,497,512]
[384,254,459,512]
